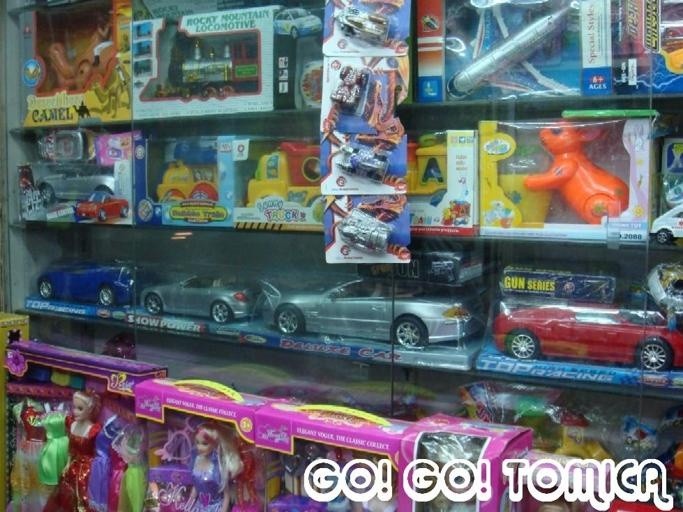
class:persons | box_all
[247,142,321,207]
[62,390,101,512]
[35,258,150,305]
[37,167,115,205]
[409,143,446,196]
[652,204,683,243]
[338,147,388,180]
[138,272,262,323]
[325,65,370,106]
[337,8,390,43]
[13,398,68,511]
[276,279,476,348]
[495,302,683,372]
[455,7,570,94]
[526,122,628,219]
[183,426,230,511]
[89,413,147,511]
[157,160,218,202]
[169,36,260,96]
[46,20,117,91]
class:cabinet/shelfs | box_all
[0,1,683,512]
